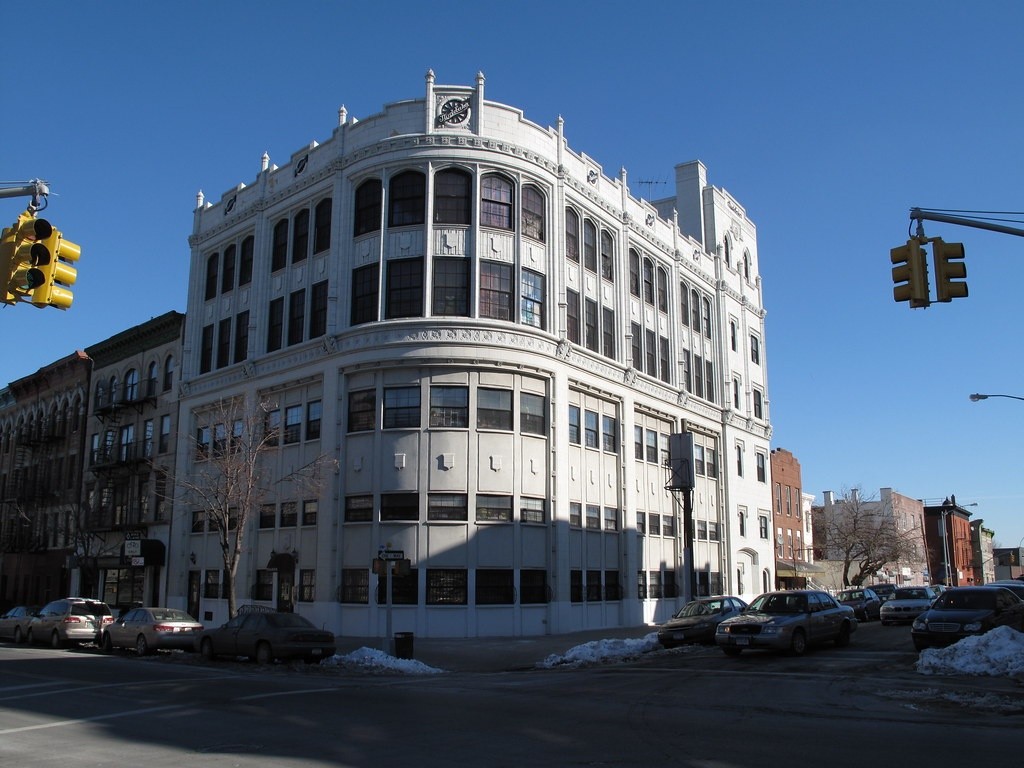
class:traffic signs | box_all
[378,550,404,562]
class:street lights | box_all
[941,502,979,586]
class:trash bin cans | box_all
[394,632,414,660]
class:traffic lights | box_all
[6,212,53,298]
[1010,555,1015,563]
[371,558,386,577]
[932,238,969,302]
[890,239,930,309]
[31,225,81,312]
[394,558,412,577]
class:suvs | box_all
[27,597,115,649]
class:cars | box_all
[910,585,1024,652]
[656,596,758,649]
[0,605,44,644]
[879,585,938,626]
[192,611,337,672]
[834,588,882,622]
[924,575,1024,602]
[866,583,900,605]
[714,590,859,658]
[101,607,205,658]
